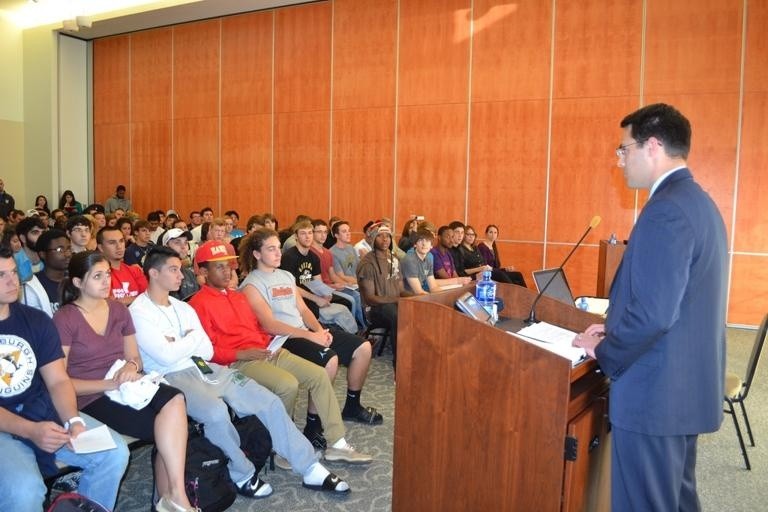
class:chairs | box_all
[723,316,767,470]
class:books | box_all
[506,321,602,370]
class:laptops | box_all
[531,264,576,309]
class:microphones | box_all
[522,213,604,324]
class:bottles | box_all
[476,271,497,313]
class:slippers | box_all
[305,431,328,450]
[235,472,274,498]
[341,404,385,425]
[301,473,353,496]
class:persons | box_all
[571,102,729,512]
[0,179,515,511]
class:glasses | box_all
[46,247,73,254]
[616,139,662,156]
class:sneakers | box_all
[326,445,374,465]
[274,455,292,471]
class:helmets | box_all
[151,409,272,511]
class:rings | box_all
[578,336,582,341]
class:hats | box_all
[194,239,240,265]
[365,219,394,252]
[162,228,193,247]
